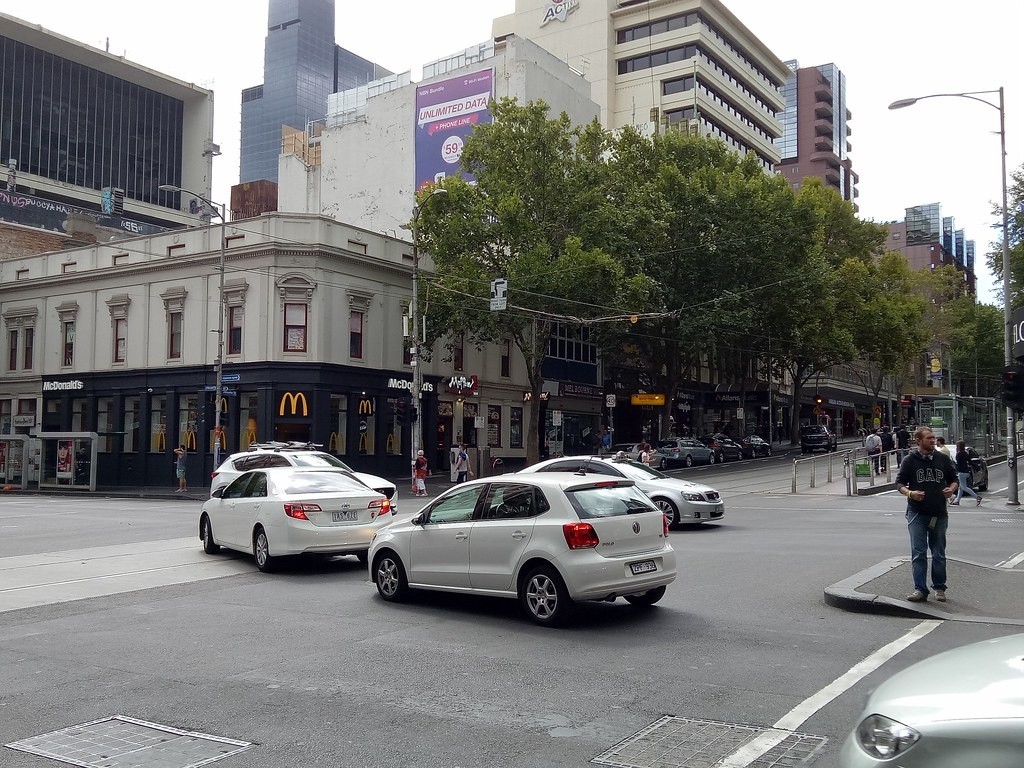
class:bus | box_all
[900,394,921,425]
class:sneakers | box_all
[934,589,946,602]
[907,590,927,602]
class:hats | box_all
[417,450,424,455]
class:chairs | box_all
[499,489,528,517]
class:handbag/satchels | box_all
[637,451,644,463]
[412,479,418,492]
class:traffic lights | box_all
[1002,366,1017,407]
[816,397,823,403]
[395,402,406,425]
[408,404,417,421]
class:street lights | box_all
[407,187,448,492]
[887,85,1016,499]
[161,185,223,473]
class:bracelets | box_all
[909,491,912,498]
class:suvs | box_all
[698,433,743,463]
[657,437,715,467]
[801,424,838,453]
[210,438,401,515]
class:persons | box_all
[896,425,912,469]
[866,428,883,475]
[174,445,188,492]
[58,441,68,472]
[951,440,982,506]
[593,430,610,455]
[936,437,950,458]
[454,445,474,483]
[879,426,895,472]
[413,450,428,497]
[894,426,959,602]
[638,442,657,466]
[778,423,783,444]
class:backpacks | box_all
[865,434,878,453]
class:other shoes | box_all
[416,493,419,496]
[976,496,982,506]
[174,489,187,493]
[420,493,428,496]
[949,502,959,507]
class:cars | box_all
[733,435,772,459]
[602,442,667,470]
[497,455,726,529]
[367,469,677,629]
[199,466,394,573]
[875,425,919,444]
[935,444,988,495]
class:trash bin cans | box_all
[478,445,491,478]
